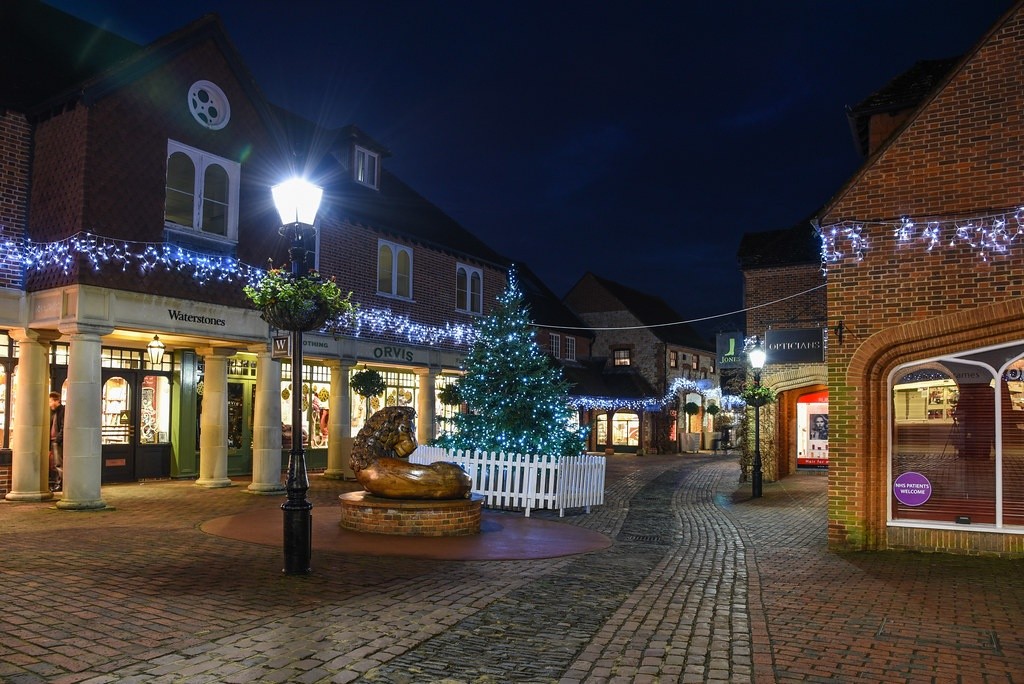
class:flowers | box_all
[243,257,354,321]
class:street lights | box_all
[267,158,325,578]
[747,335,767,499]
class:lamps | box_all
[145,334,165,364]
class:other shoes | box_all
[51,484,62,492]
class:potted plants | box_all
[741,386,777,409]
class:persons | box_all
[49,392,66,492]
[809,414,829,440]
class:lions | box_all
[350,406,473,501]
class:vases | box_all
[261,297,328,332]
